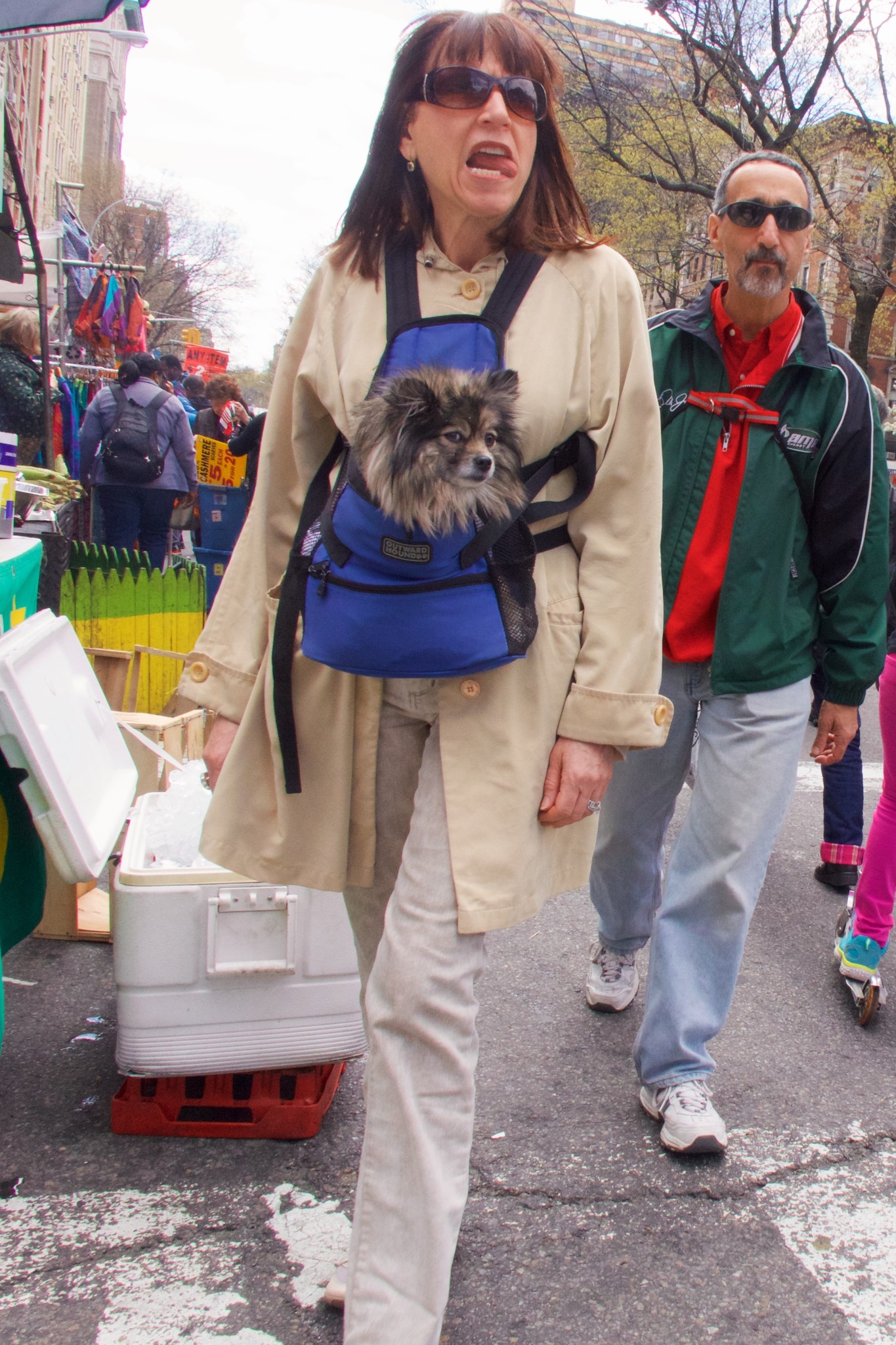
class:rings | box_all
[200,772,210,790]
[587,800,602,811]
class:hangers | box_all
[49,263,132,384]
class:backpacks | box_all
[101,384,173,484]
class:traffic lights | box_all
[181,328,200,348]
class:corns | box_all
[17,465,84,509]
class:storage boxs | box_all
[0,483,367,1142]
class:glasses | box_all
[407,66,547,121]
[716,202,815,231]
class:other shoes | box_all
[814,863,857,886]
[325,1264,343,1303]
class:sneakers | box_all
[639,1080,729,1154]
[833,928,889,981]
[586,941,641,1010]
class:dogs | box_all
[357,368,530,537]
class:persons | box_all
[177,14,674,1345]
[3,305,347,580]
[584,150,896,1164]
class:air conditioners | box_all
[63,41,89,90]
[7,91,17,104]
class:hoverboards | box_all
[832,858,888,1028]
